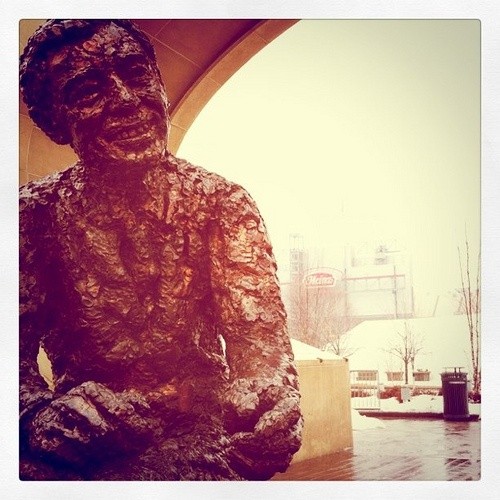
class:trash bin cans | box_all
[441,366,471,422]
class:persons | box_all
[19,17,306,480]
